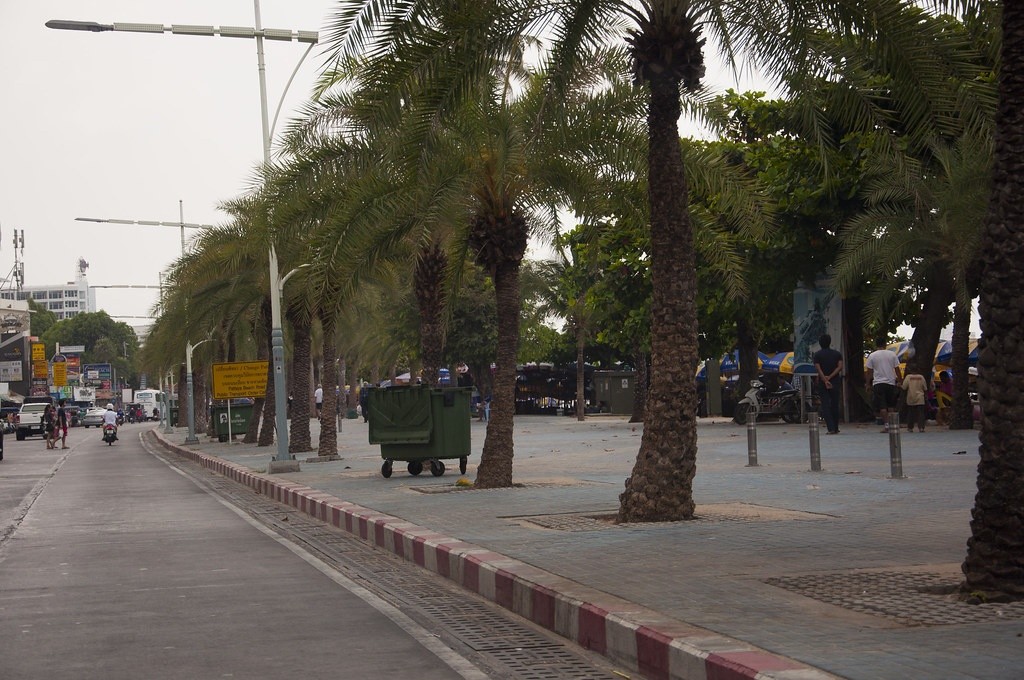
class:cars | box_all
[0,411,8,461]
[81,410,106,428]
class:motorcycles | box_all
[118,416,124,426]
[137,416,141,423]
[102,417,119,446]
[153,415,159,422]
[734,379,801,425]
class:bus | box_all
[134,390,166,421]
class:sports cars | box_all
[129,417,134,424]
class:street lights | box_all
[74,198,213,445]
[44,19,320,474]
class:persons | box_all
[939,371,954,398]
[866,336,900,425]
[51,406,58,421]
[103,403,118,427]
[777,374,793,390]
[52,399,70,449]
[65,410,71,428]
[117,408,124,419]
[360,381,380,423]
[44,404,58,449]
[476,390,485,423]
[315,384,323,420]
[129,407,159,419]
[696,377,708,417]
[813,335,843,435]
[897,363,927,433]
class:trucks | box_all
[23,396,56,408]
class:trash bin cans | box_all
[209,403,254,442]
[367,386,472,478]
[170,408,178,427]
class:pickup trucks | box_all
[15,403,51,441]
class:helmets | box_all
[107,404,113,410]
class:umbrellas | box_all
[695,336,981,418]
[380,361,471,388]
[331,384,359,396]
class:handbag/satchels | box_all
[58,429,64,437]
[45,424,54,433]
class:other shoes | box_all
[363,419,367,423]
[318,415,321,420]
[47,446,53,449]
[884,423,890,431]
[53,446,58,448]
[825,430,841,434]
[50,440,54,449]
[62,446,70,449]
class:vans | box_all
[55,406,86,418]
